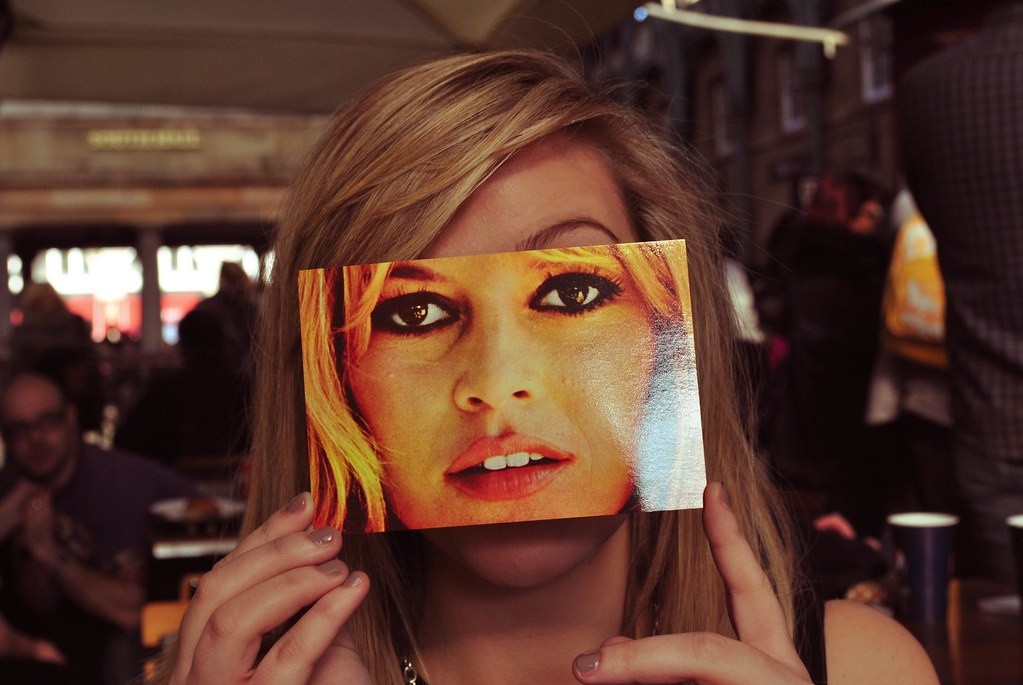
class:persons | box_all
[0,265,260,685]
[296,240,707,536]
[155,49,944,685]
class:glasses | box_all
[3,400,71,444]
